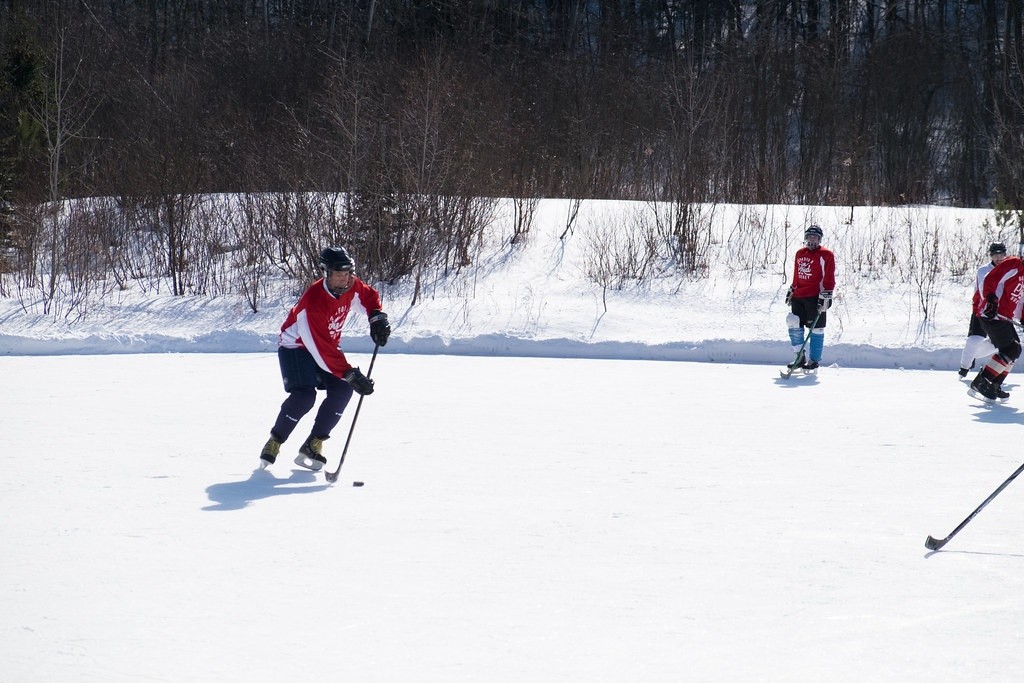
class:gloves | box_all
[1020,320,1024,332]
[983,292,998,319]
[785,286,797,306]
[817,290,833,311]
[368,310,391,346]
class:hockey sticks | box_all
[780,311,821,379]
[324,334,381,483]
[925,463,1024,550]
[995,313,1024,328]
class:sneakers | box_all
[341,366,375,395]
[802,357,822,374]
[295,431,330,470]
[994,380,1010,403]
[259,433,281,469]
[968,366,998,404]
[969,358,975,372]
[787,349,807,374]
[958,367,969,380]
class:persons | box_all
[967,248,1024,403]
[260,247,391,470]
[958,242,1006,381]
[785,225,835,374]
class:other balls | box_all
[352,481,365,487]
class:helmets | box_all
[319,245,355,294]
[989,241,1007,260]
[804,225,823,250]
